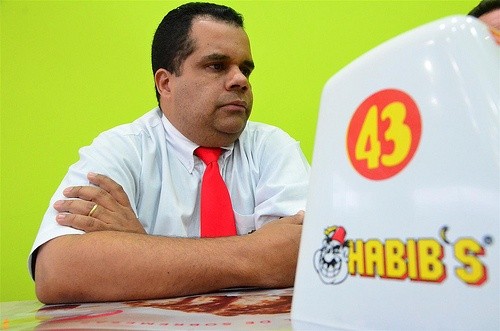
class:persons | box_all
[28,2,311,304]
[467,0,500,32]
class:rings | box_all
[88,204,97,216]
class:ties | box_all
[195,147,236,236]
